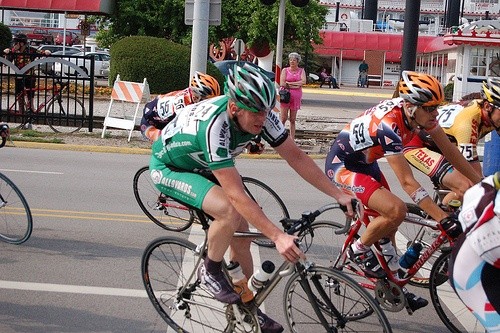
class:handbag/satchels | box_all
[279,83,290,103]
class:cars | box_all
[27,40,111,79]
[211,60,276,85]
[56,31,80,46]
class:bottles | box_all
[378,237,400,272]
[399,243,423,270]
[247,260,275,292]
[227,260,254,302]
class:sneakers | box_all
[239,303,284,333]
[394,287,428,308]
[346,243,386,279]
[198,263,241,304]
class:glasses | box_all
[421,105,438,113]
[14,42,22,45]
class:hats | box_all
[10,34,27,42]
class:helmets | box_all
[480,77,500,109]
[224,63,277,114]
[399,70,444,106]
[190,71,220,99]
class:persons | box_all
[4,34,52,129]
[141,71,264,155]
[402,74,500,333]
[0,123,10,137]
[325,71,483,311]
[280,52,306,141]
[149,63,363,333]
[359,60,369,88]
[321,68,339,89]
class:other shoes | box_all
[28,124,32,130]
[16,125,26,130]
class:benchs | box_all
[319,72,331,88]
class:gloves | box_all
[246,140,264,155]
[440,216,462,238]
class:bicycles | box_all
[140,198,393,333]
[295,176,490,333]
[0,125,34,245]
[0,71,87,135]
[132,164,290,248]
